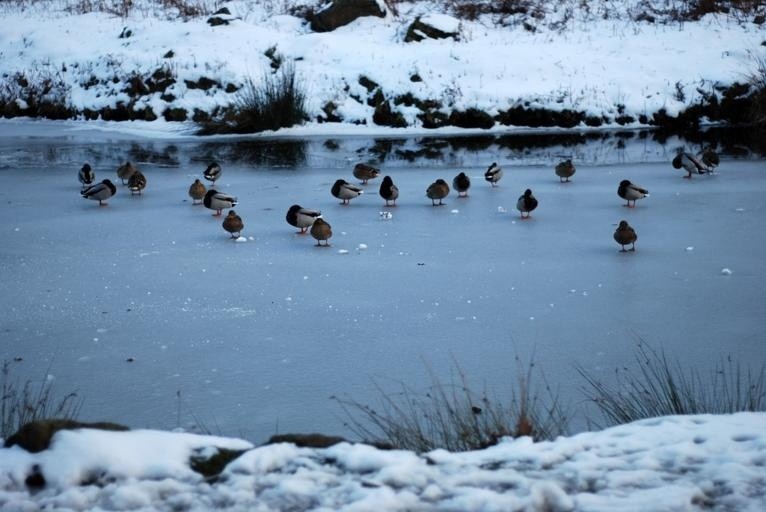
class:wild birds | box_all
[616,179,649,208]
[285,204,323,234]
[78,160,147,208]
[612,220,638,252]
[484,162,503,186]
[516,189,538,219]
[426,172,450,206]
[452,172,470,198]
[311,218,333,247]
[555,159,576,183]
[189,161,244,240]
[331,162,400,208]
[702,145,720,174]
[672,146,709,179]
